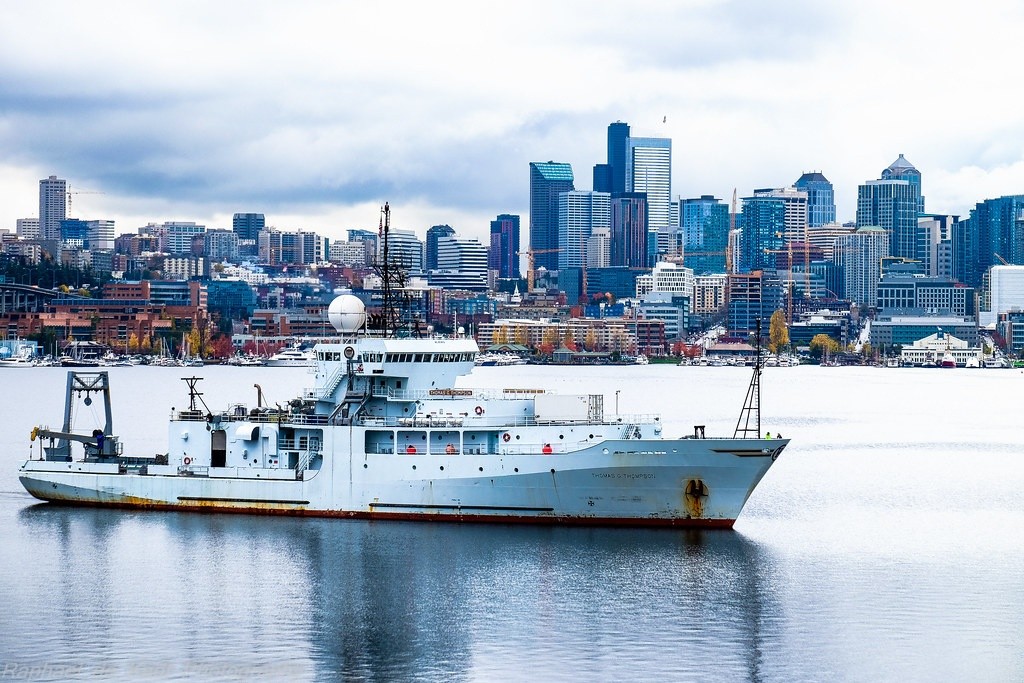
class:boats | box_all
[904,361,914,367]
[820,357,841,367]
[680,344,800,367]
[921,350,936,367]
[965,357,1003,368]
[0,332,203,368]
[532,322,649,365]
[19,201,791,531]
[475,350,530,366]
[227,316,322,367]
[941,334,957,368]
[887,355,898,368]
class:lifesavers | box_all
[475,406,482,416]
[184,457,191,465]
[360,364,363,371]
[503,433,511,443]
